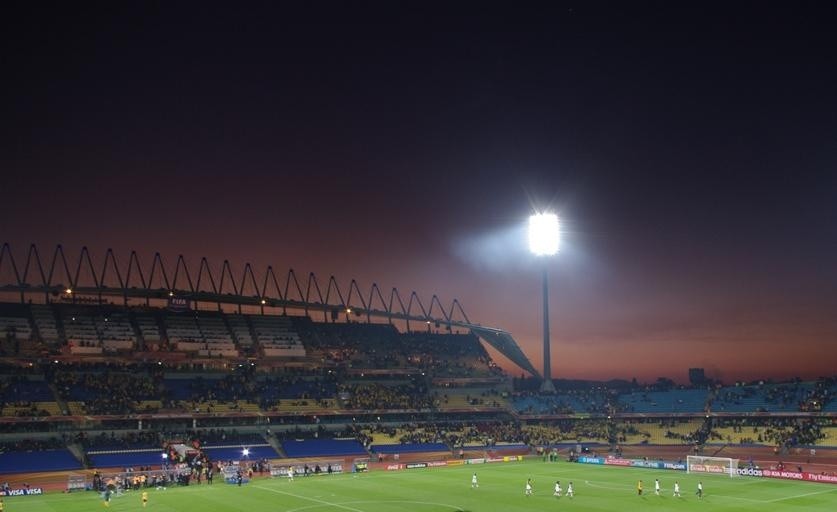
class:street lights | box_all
[524,212,560,395]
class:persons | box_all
[105,490,111,508]
[0,500,3,512]
[142,491,148,507]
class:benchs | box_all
[0,378,837,450]
[0,311,309,359]
